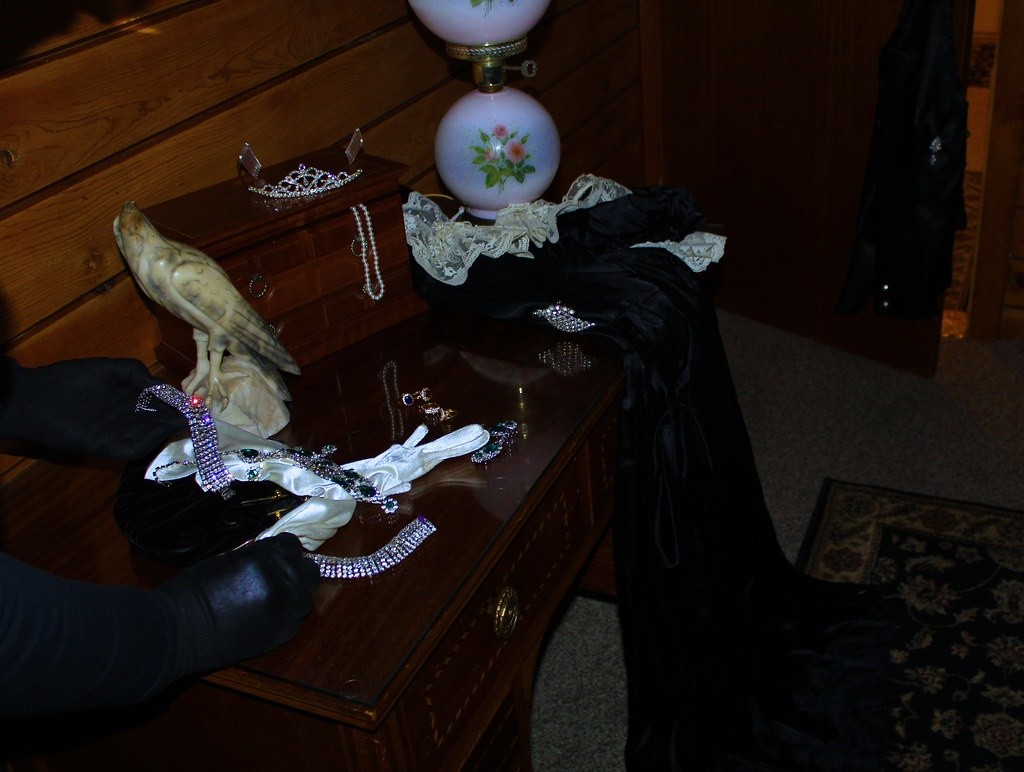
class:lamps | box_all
[408,0,561,220]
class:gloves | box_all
[0,356,188,460]
[157,532,320,688]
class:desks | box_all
[122,313,631,772]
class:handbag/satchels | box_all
[116,438,307,568]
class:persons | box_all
[0,531,320,726]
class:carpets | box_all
[794,475,1024,772]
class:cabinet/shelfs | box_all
[141,146,426,377]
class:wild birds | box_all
[112,202,303,415]
